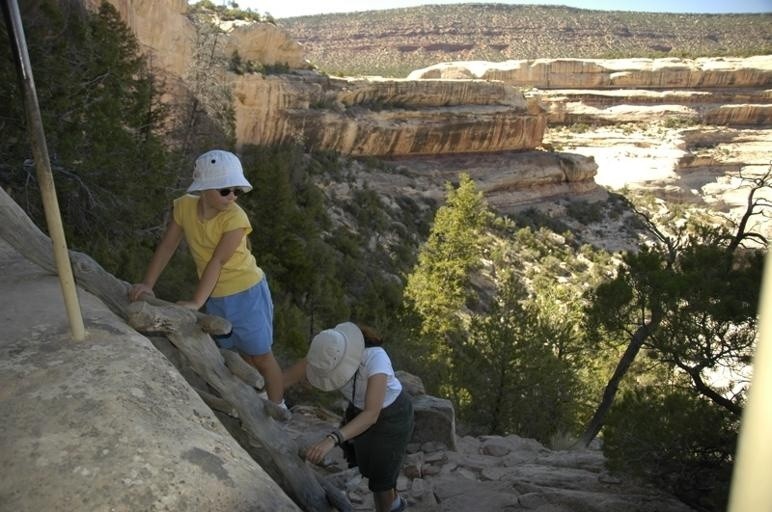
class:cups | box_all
[216,188,242,197]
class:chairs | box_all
[391,496,408,512]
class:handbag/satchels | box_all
[326,430,344,447]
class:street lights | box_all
[339,402,358,469]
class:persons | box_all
[126,146,290,413]
[282,319,415,511]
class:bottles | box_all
[305,321,366,392]
[186,150,253,197]
